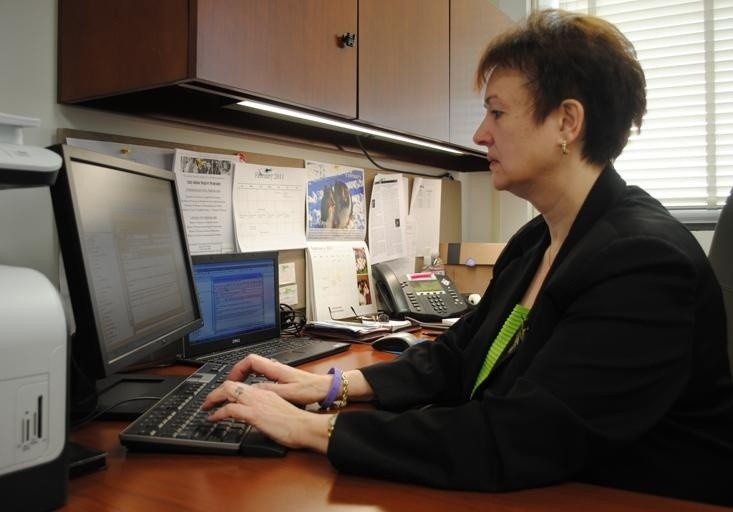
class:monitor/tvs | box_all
[44,144,204,421]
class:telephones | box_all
[371,263,468,320]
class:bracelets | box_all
[332,369,349,410]
[328,413,338,436]
[321,367,342,410]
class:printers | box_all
[0,114,67,512]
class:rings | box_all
[234,386,244,402]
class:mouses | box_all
[371,332,417,351]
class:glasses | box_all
[328,304,389,326]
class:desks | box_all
[60,318,732,512]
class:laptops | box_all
[181,250,351,378]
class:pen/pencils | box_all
[422,333,441,336]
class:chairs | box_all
[708,186,733,373]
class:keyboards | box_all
[118,361,278,457]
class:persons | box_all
[200,7,733,506]
[355,250,367,274]
[358,280,370,305]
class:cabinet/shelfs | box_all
[191,1,516,155]
